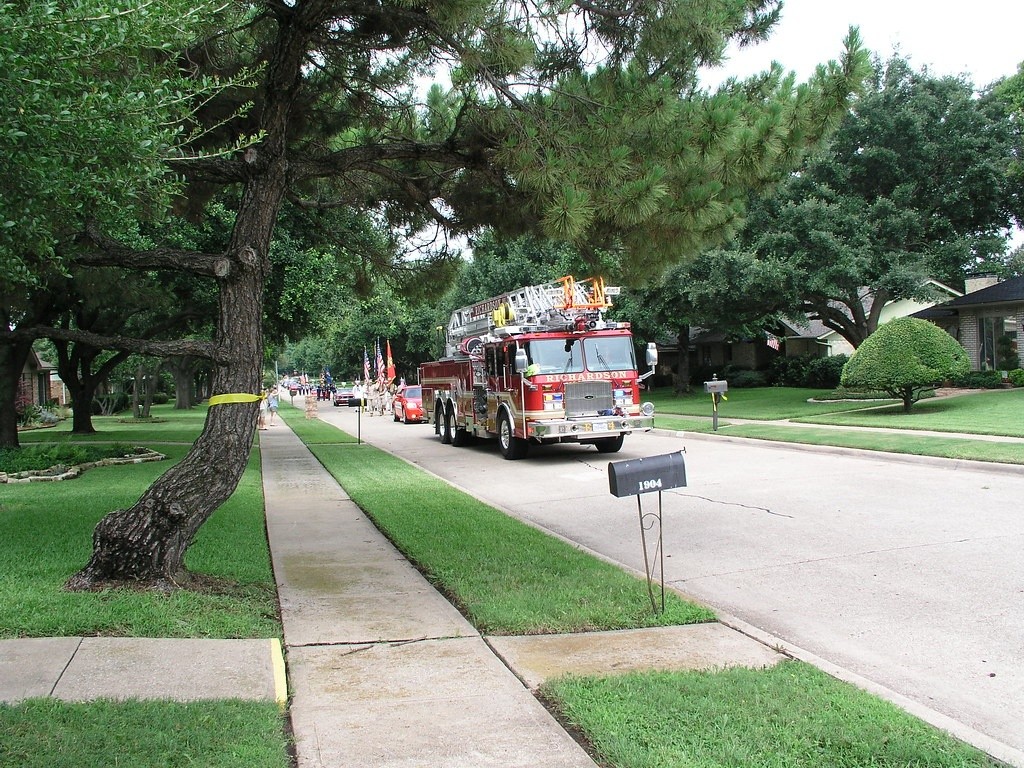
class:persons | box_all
[259,388,282,428]
[317,384,336,401]
[353,380,407,416]
[297,385,315,395]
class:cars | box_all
[392,385,424,424]
[281,378,302,391]
[333,388,355,406]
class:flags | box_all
[387,344,396,385]
[767,334,779,351]
[364,353,371,383]
[373,344,386,385]
[322,368,331,384]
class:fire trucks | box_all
[421,273,658,460]
